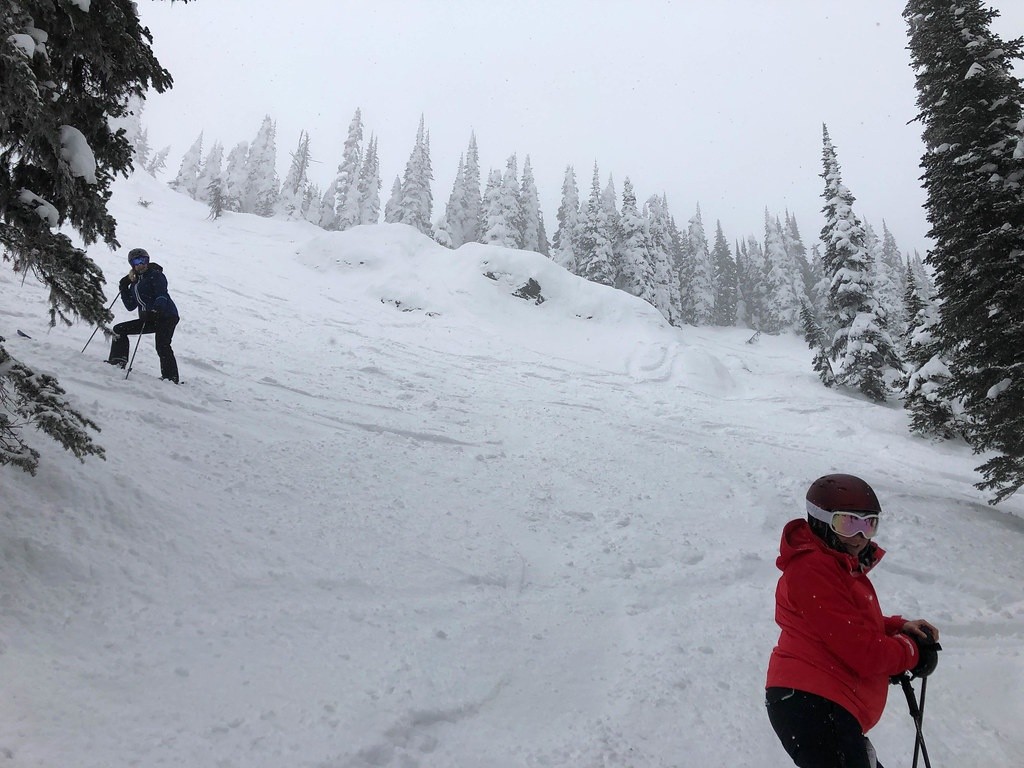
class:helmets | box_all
[128,248,149,263]
[807,473,881,514]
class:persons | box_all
[765,474,942,768]
[102,249,180,383]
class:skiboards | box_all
[16,329,187,386]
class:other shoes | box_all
[104,357,126,369]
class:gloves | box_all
[120,274,132,286]
[140,311,155,322]
[906,626,941,677]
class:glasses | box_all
[132,256,150,265]
[829,512,880,540]
